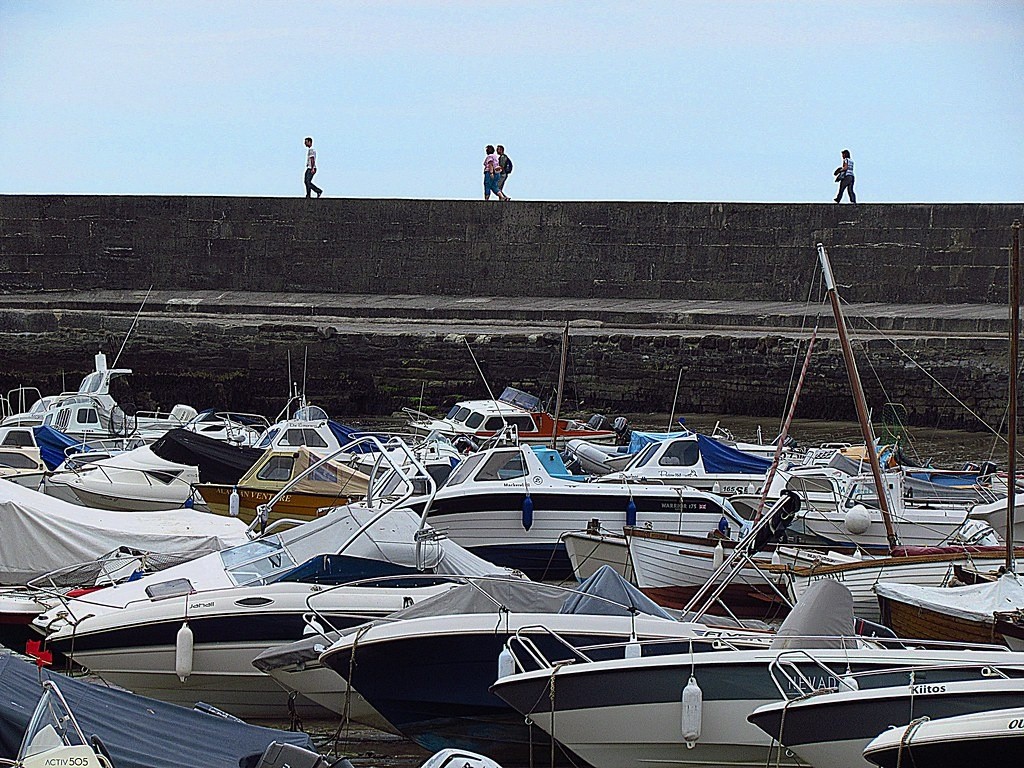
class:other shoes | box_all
[499,198,506,201]
[506,197,511,201]
[834,198,840,203]
[316,190,323,198]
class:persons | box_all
[834,150,856,203]
[482,145,511,201]
[304,138,323,198]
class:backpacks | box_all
[505,159,513,173]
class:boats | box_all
[0,351,1024,768]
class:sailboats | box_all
[729,240,1024,633]
[876,221,1024,652]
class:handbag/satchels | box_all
[494,166,502,173]
